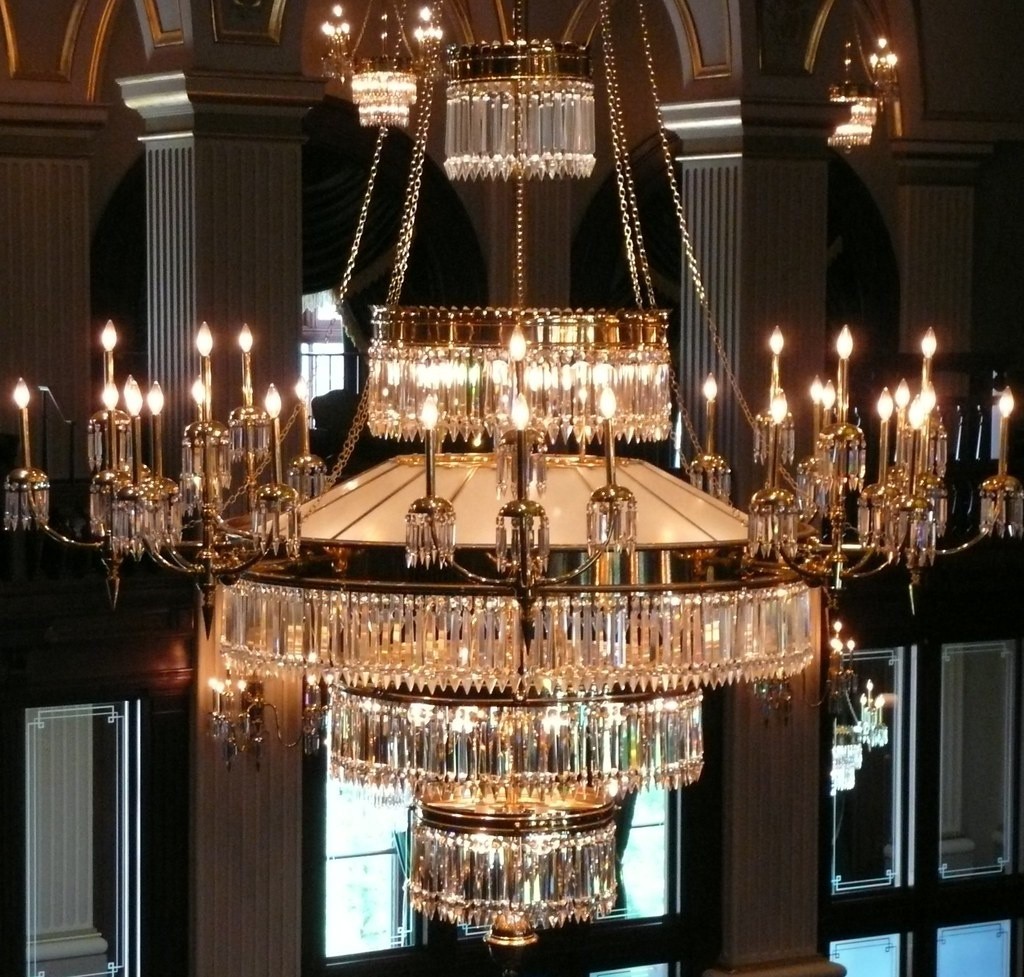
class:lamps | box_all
[6,0,1024,944]
